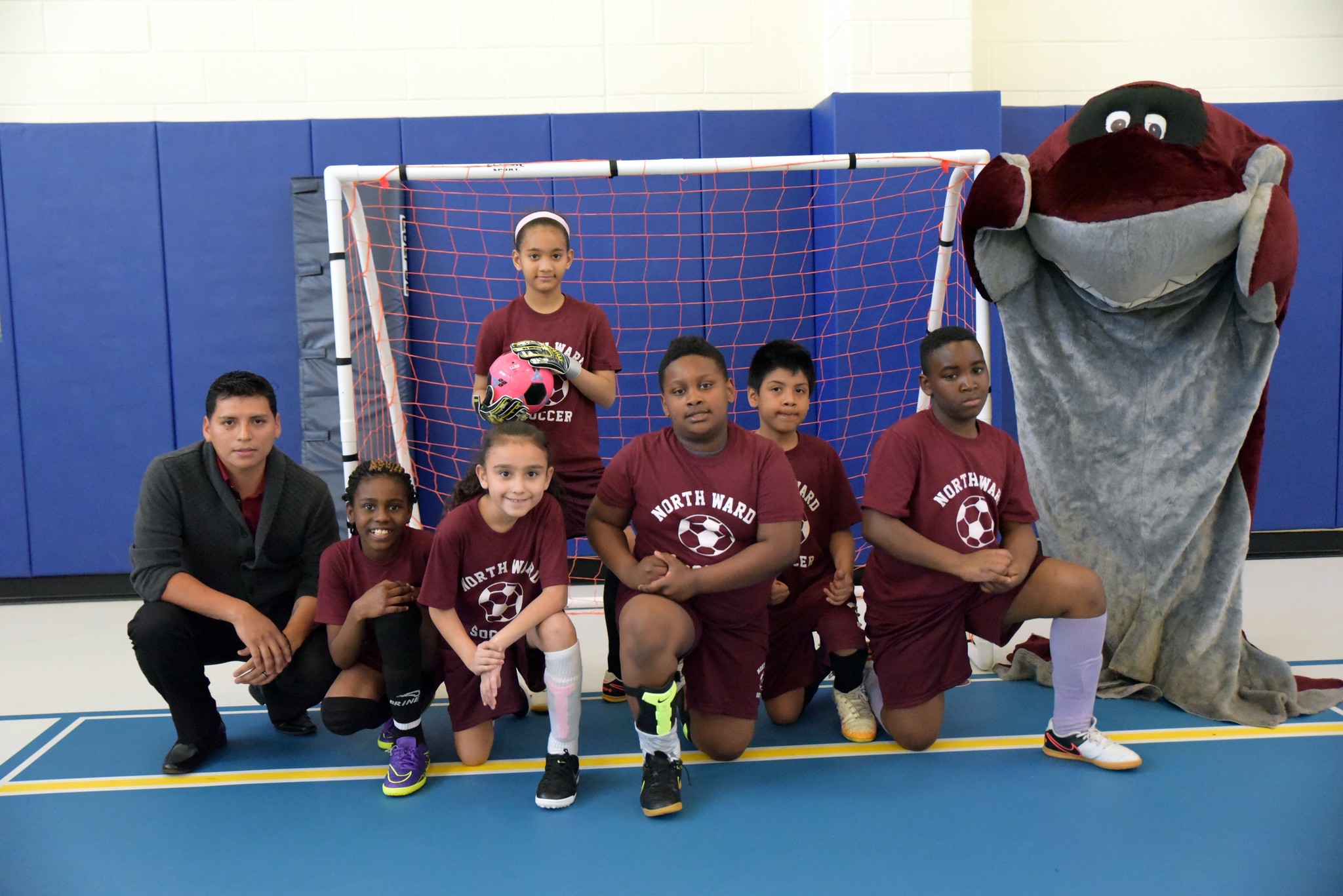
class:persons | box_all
[746,342,877,743]
[126,370,342,776]
[317,458,443,795]
[586,335,804,816]
[416,424,583,808]
[472,212,635,710]
[861,327,1141,770]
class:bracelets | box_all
[281,631,292,657]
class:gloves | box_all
[511,341,581,381]
[475,385,529,427]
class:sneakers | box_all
[248,683,317,737]
[378,718,398,749]
[383,737,430,797]
[640,751,690,818]
[162,732,228,774]
[1043,714,1142,769]
[832,683,876,742]
[535,749,580,808]
[602,671,628,702]
[675,663,689,739]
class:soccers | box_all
[487,351,554,415]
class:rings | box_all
[261,671,269,678]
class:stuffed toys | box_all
[959,82,1343,730]
